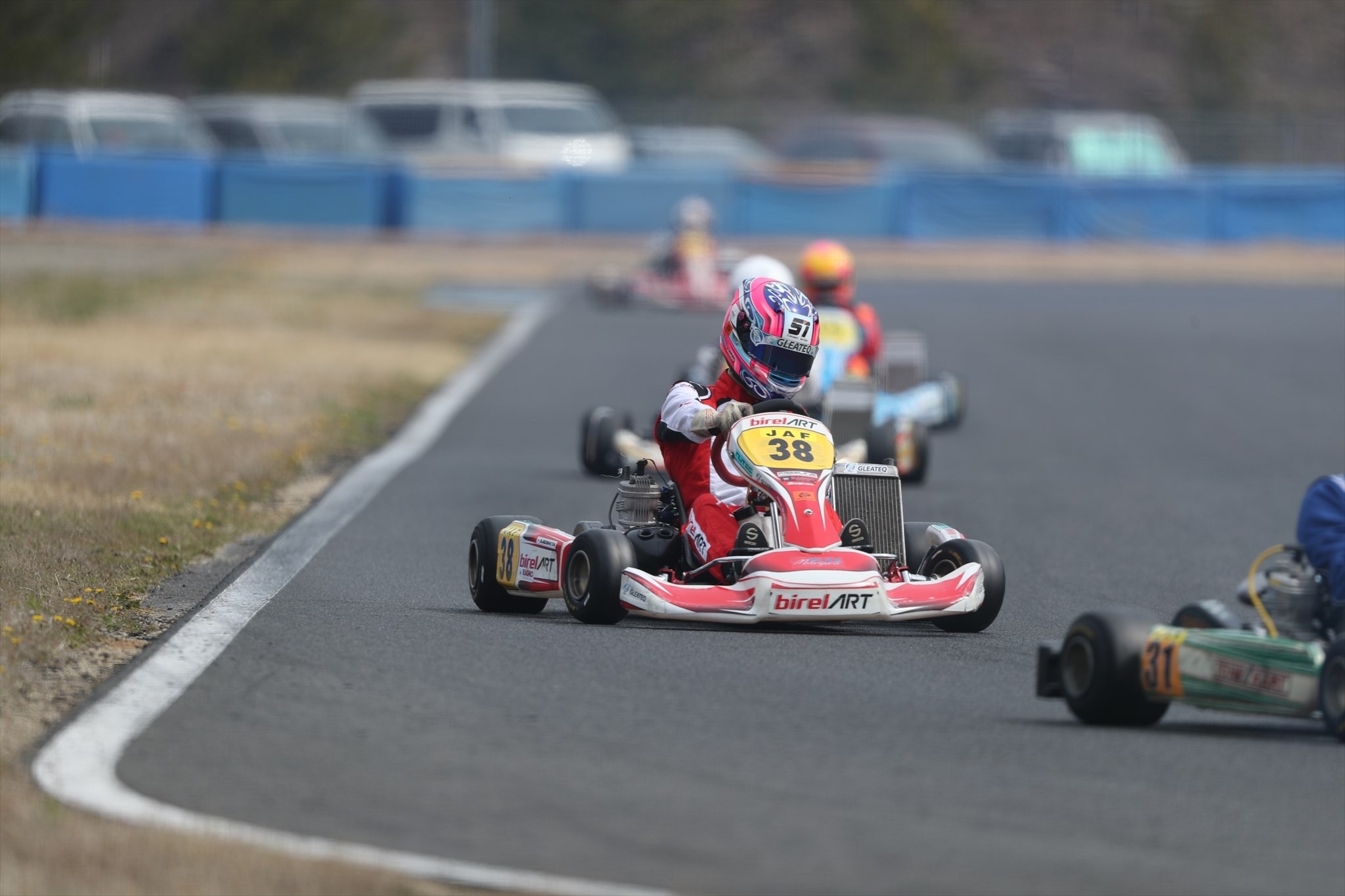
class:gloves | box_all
[690,400,754,441]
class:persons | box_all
[645,198,719,277]
[1296,472,1345,641]
[653,278,874,584]
[689,239,880,387]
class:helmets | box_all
[799,240,856,307]
[727,254,794,303]
[719,277,819,399]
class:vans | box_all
[348,74,638,175]
[0,88,382,165]
[972,106,1194,173]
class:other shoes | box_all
[838,518,870,547]
[733,522,769,575]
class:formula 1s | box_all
[1035,543,1344,738]
[464,304,1007,634]
[623,231,757,310]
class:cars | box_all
[782,111,1003,170]
[632,118,774,169]
